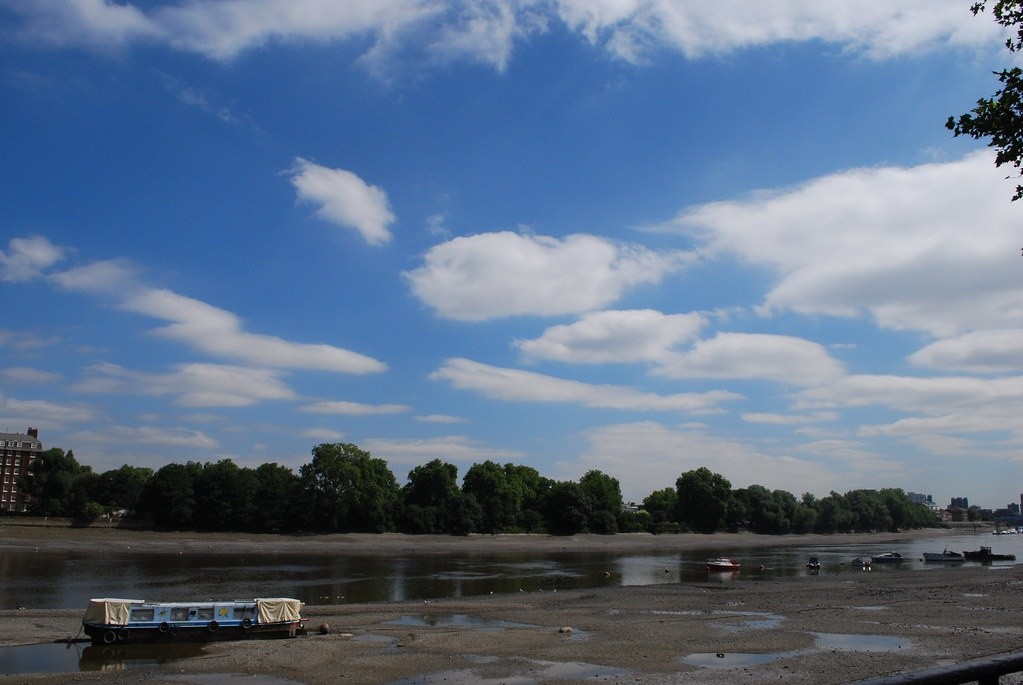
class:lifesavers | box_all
[241,618,252,629]
[158,622,170,634]
[170,625,181,637]
[117,628,131,641]
[208,620,219,633]
[103,630,117,644]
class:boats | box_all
[922,545,966,561]
[963,541,1020,560]
[78,594,308,644]
[871,546,903,564]
[706,555,743,571]
[807,553,822,575]
[992,528,1023,534]
